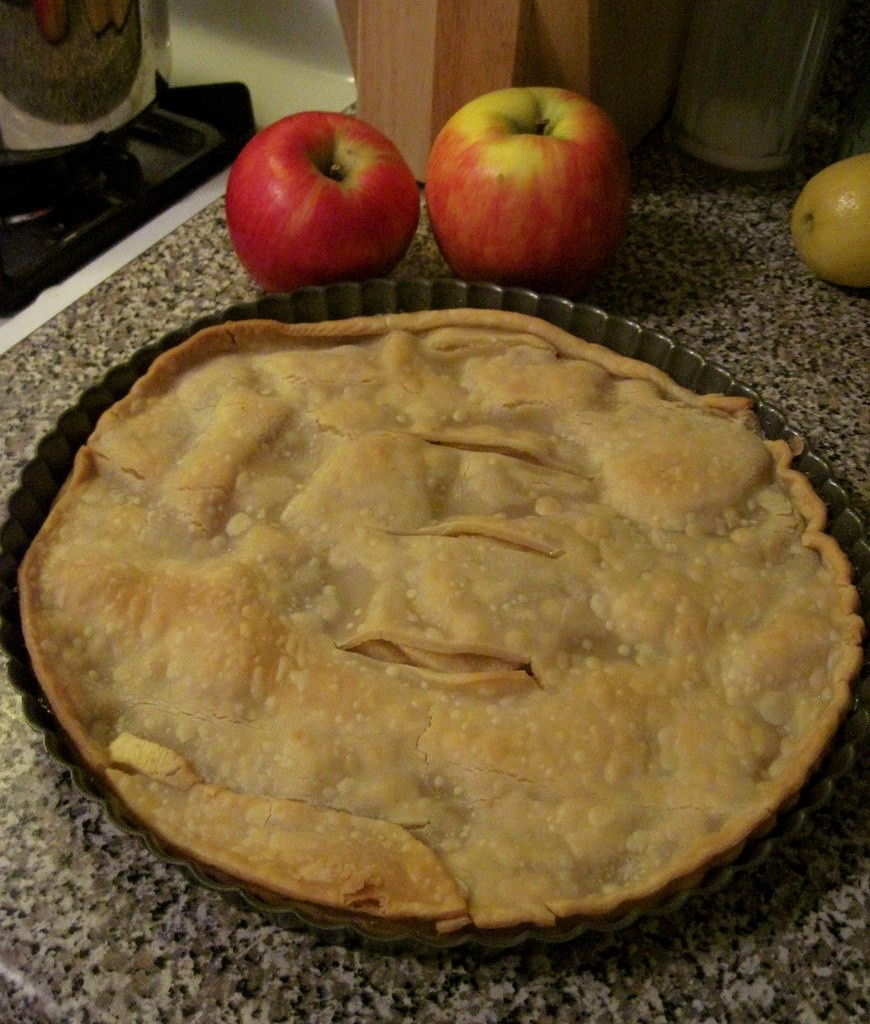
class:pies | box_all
[17,308,862,934]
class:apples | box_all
[225,110,421,294]
[424,86,628,296]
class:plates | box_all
[0,278,870,962]
